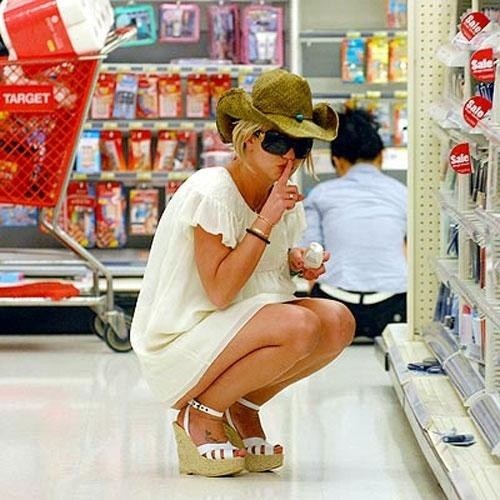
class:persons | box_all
[126,65,356,478]
[298,102,409,340]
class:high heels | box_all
[172,398,246,478]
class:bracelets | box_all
[250,225,269,239]
[255,213,275,227]
[288,247,300,276]
[246,228,271,244]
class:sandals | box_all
[224,397,285,472]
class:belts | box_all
[321,281,399,306]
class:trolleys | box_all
[0,25,139,352]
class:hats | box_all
[215,69,340,144]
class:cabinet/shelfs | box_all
[4,0,303,333]
[408,0,500,464]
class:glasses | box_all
[256,126,314,158]
